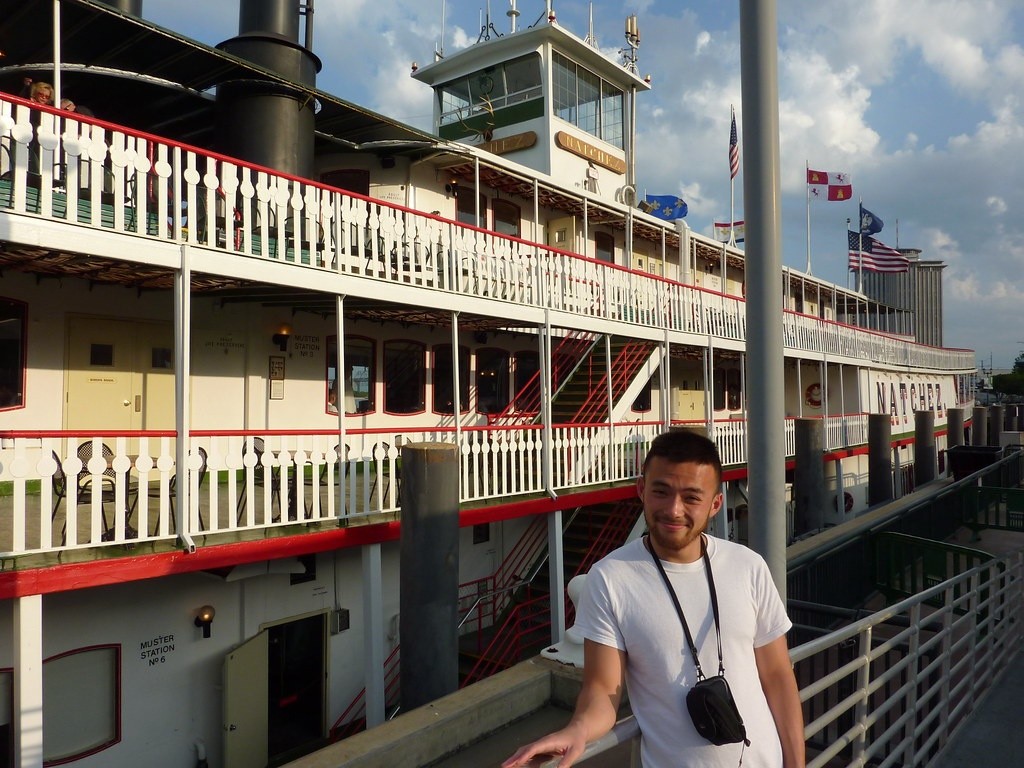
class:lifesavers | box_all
[805,383,829,406]
[833,492,853,513]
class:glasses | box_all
[64,104,71,110]
[37,92,50,100]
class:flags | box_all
[859,199,885,234]
[847,228,910,272]
[647,194,690,219]
[729,109,740,178]
[714,220,746,243]
[806,167,853,202]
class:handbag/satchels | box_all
[686,675,750,746]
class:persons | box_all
[23,82,55,160]
[328,389,340,413]
[332,362,359,414]
[501,428,809,768]
[61,98,95,139]
[3,69,39,101]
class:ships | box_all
[1,0,1023,768]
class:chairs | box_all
[49,436,413,548]
[1,134,472,294]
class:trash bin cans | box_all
[942,445,1004,506]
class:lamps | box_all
[195,605,215,639]
[274,325,289,350]
[450,180,458,197]
